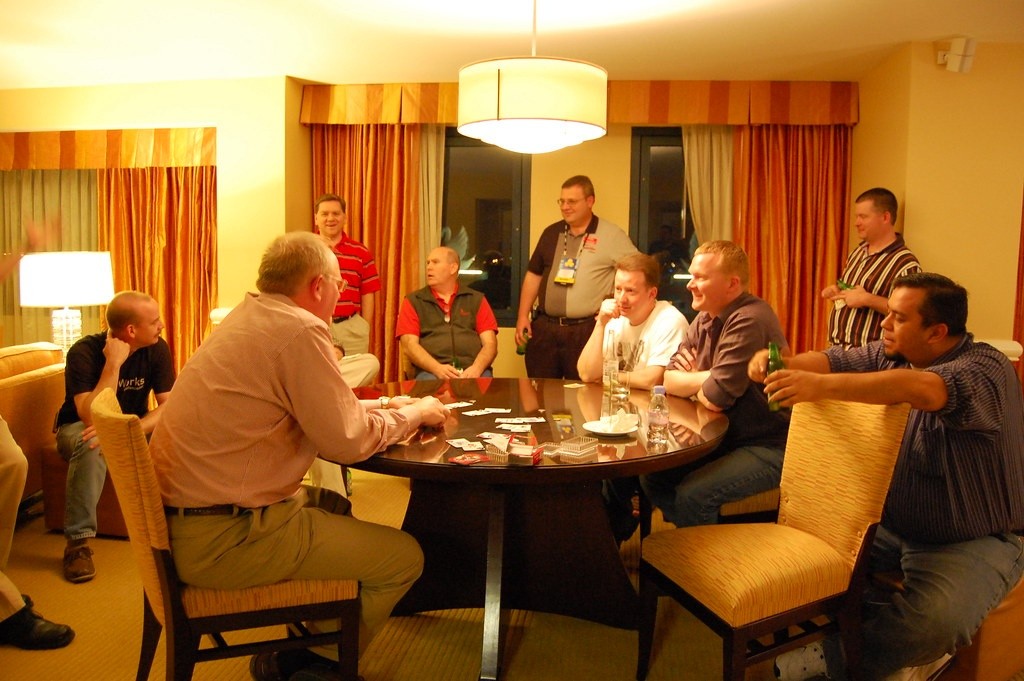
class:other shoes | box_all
[251,643,365,681]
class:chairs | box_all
[719,485,780,514]
[636,399,917,680]
[91,387,361,680]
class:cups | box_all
[610,370,631,403]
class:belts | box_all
[331,312,356,323]
[161,505,267,516]
[540,312,594,326]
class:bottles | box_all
[603,330,619,386]
[767,341,785,411]
[647,386,669,443]
[516,329,528,355]
[836,280,853,290]
[347,469,353,495]
[452,358,461,370]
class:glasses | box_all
[557,197,582,206]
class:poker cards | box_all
[444,416,546,466]
[563,383,586,389]
[443,401,512,416]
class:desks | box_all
[316,377,729,681]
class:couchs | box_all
[0,341,66,525]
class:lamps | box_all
[19,251,115,357]
[458,2,609,153]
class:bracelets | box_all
[379,396,390,409]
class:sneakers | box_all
[773,638,826,681]
[882,652,954,681]
[62,544,96,582]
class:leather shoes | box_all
[0,595,75,651]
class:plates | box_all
[582,420,638,437]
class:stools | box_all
[44,435,133,540]
[875,562,1024,681]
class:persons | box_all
[520,377,589,464]
[664,393,729,445]
[748,273,1024,681]
[401,379,492,437]
[313,191,382,354]
[148,232,452,680]
[577,382,640,440]
[307,353,380,499]
[0,419,76,652]
[581,257,690,551]
[516,175,642,382]
[55,290,175,583]
[822,188,922,351]
[647,241,793,530]
[395,246,497,380]
